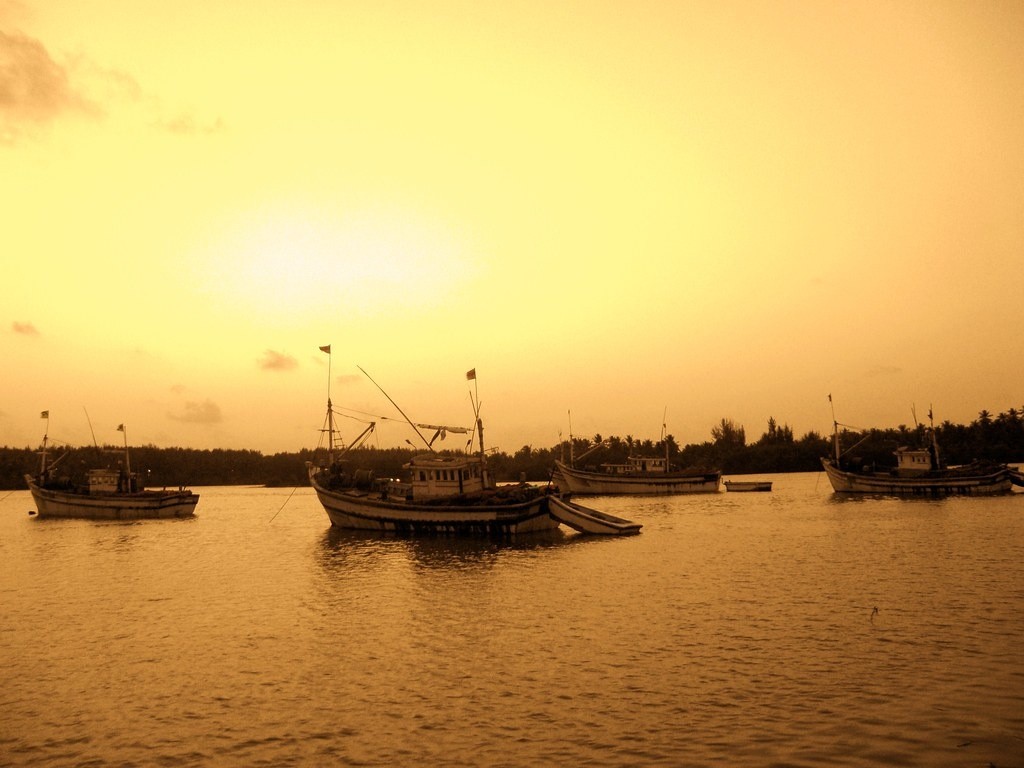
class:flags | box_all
[116,423,123,431]
[40,411,48,418]
[319,346,330,354]
[466,369,476,380]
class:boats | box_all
[23,411,200,520]
[724,480,773,493]
[1007,468,1024,487]
[820,392,1018,494]
[547,408,722,496]
[304,345,570,537]
[547,494,644,536]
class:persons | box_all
[35,475,41,486]
